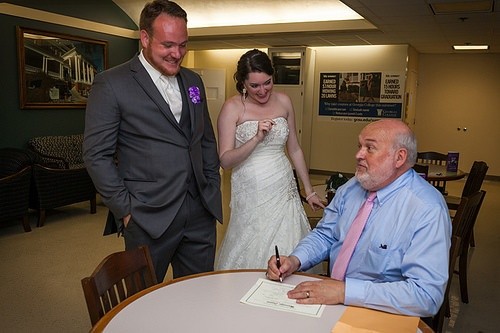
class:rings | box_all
[266,273,268,276]
[306,291,309,298]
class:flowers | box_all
[188,84,200,104]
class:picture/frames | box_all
[16,25,109,110]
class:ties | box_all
[331,191,376,282]
[160,75,182,123]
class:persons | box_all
[214,49,327,271]
[84,0,222,289]
[266,118,452,316]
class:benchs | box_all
[27,135,119,171]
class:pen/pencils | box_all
[274,245,283,283]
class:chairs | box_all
[81,242,157,328]
[0,147,97,232]
[420,151,488,333]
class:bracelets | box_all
[306,191,317,201]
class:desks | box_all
[88,269,434,333]
[428,164,465,182]
